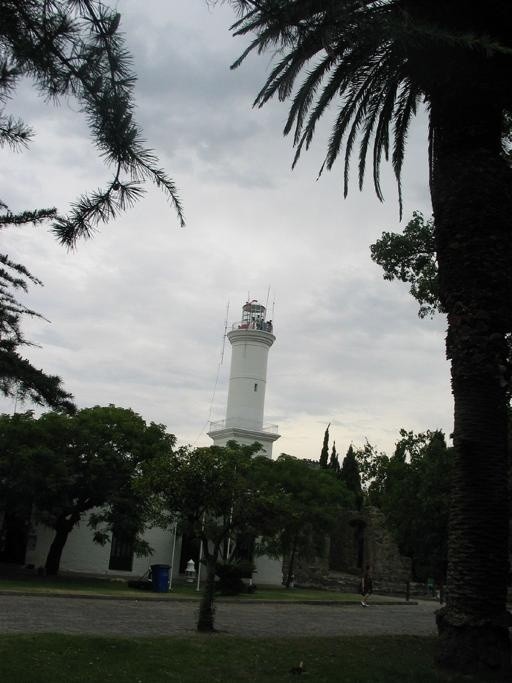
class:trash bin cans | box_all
[151,564,172,592]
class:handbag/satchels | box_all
[358,580,368,596]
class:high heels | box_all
[366,605,369,606]
[360,601,366,606]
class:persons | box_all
[248,315,274,332]
[360,564,374,606]
